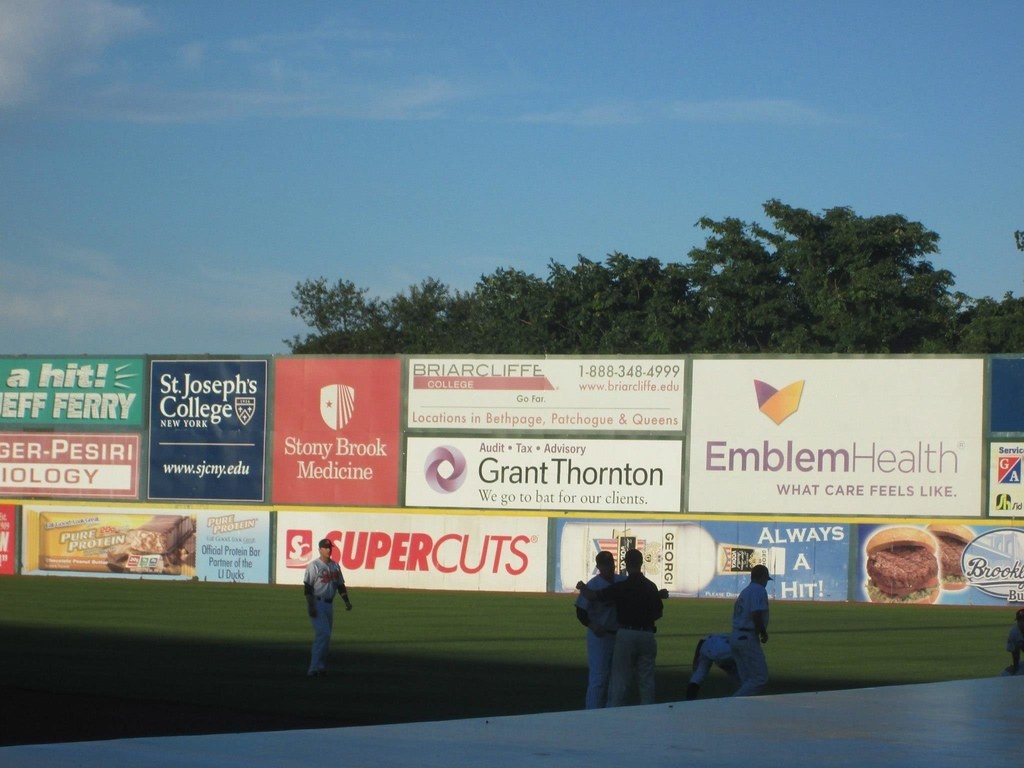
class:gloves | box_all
[308,604,316,616]
[345,602,352,611]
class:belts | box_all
[618,625,657,633]
[607,630,617,635]
[738,629,751,631]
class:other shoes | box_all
[307,670,330,680]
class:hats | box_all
[621,549,642,561]
[1014,609,1024,620]
[751,565,774,580]
[596,551,615,561]
[319,538,332,547]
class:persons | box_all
[684,634,734,701]
[574,551,668,709]
[303,539,351,682]
[576,549,664,707]
[1003,609,1024,676]
[730,564,773,696]
